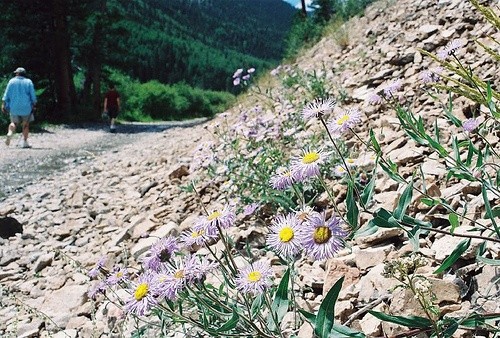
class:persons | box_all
[3,68,39,149]
[103,85,123,133]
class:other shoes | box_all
[5,132,13,145]
[23,142,32,148]
[111,128,117,133]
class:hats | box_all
[13,67,26,73]
